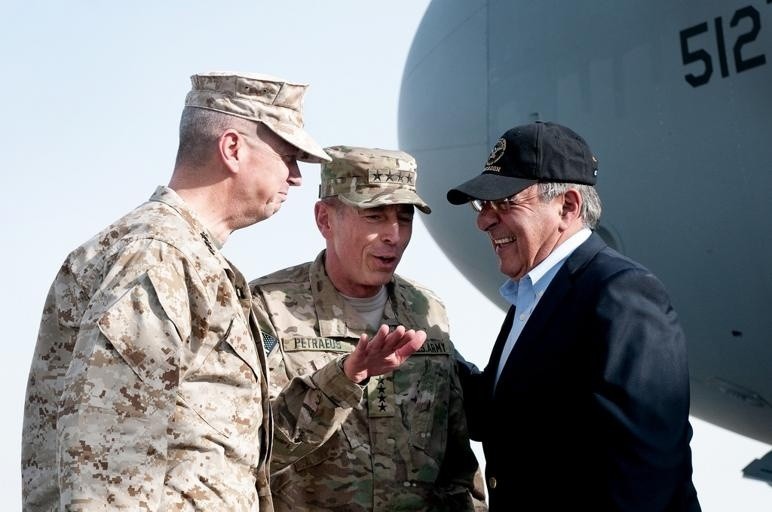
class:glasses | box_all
[469,186,565,215]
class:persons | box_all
[239,147,491,512]
[447,123,704,512]
[22,70,428,512]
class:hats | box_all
[183,70,334,163]
[448,120,598,206]
[319,145,432,214]
